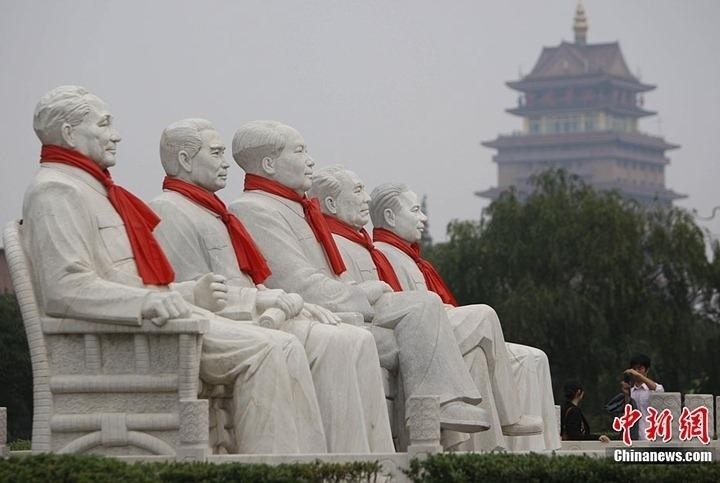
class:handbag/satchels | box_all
[562,431,569,440]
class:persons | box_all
[23,84,333,455]
[305,165,544,450]
[561,379,612,444]
[368,182,563,451]
[618,354,664,441]
[225,121,492,451]
[144,116,398,455]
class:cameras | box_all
[624,373,634,387]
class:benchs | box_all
[2,222,404,460]
